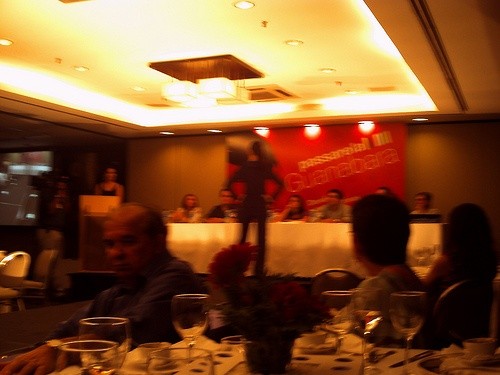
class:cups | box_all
[56,316,131,375]
[147,346,215,375]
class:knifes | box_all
[390,349,432,367]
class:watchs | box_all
[44,338,63,354]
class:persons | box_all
[314,190,352,222]
[409,191,436,215]
[0,203,201,375]
[170,194,201,222]
[325,193,429,343]
[203,189,239,222]
[280,196,313,221]
[35,170,73,291]
[425,203,497,292]
[376,186,389,194]
[225,140,286,277]
[93,167,124,201]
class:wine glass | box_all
[320,288,384,367]
[389,290,428,373]
[171,292,212,356]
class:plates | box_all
[294,344,336,354]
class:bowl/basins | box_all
[138,342,172,357]
[303,330,328,345]
[463,337,497,357]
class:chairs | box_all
[312,267,362,291]
[406,269,472,348]
[0,242,62,313]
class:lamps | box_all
[148,54,264,114]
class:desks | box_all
[166,224,450,277]
[54,327,500,375]
